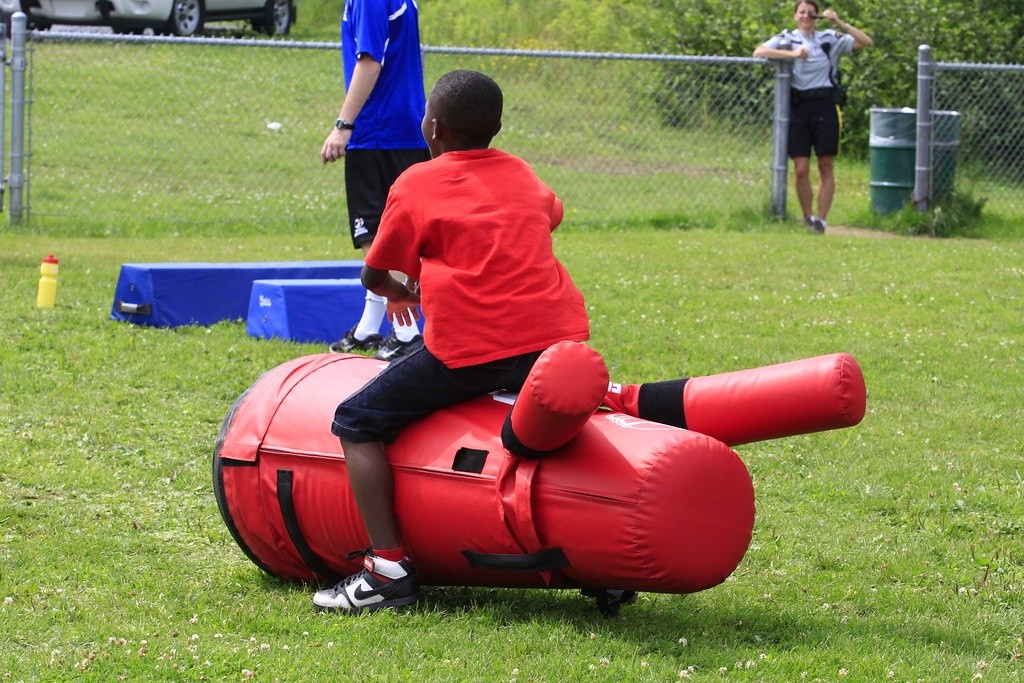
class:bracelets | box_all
[842,23,849,32]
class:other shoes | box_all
[807,220,825,235]
[806,215,815,226]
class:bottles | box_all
[34,255,59,309]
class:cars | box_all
[0,0,299,37]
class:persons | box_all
[320,1,425,362]
[313,69,639,614]
[752,1,872,234]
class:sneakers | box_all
[374,333,425,362]
[329,323,384,355]
[312,553,425,614]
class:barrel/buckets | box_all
[867,107,962,218]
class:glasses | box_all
[807,13,827,20]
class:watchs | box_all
[334,118,355,131]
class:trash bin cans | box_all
[869,106,961,215]
